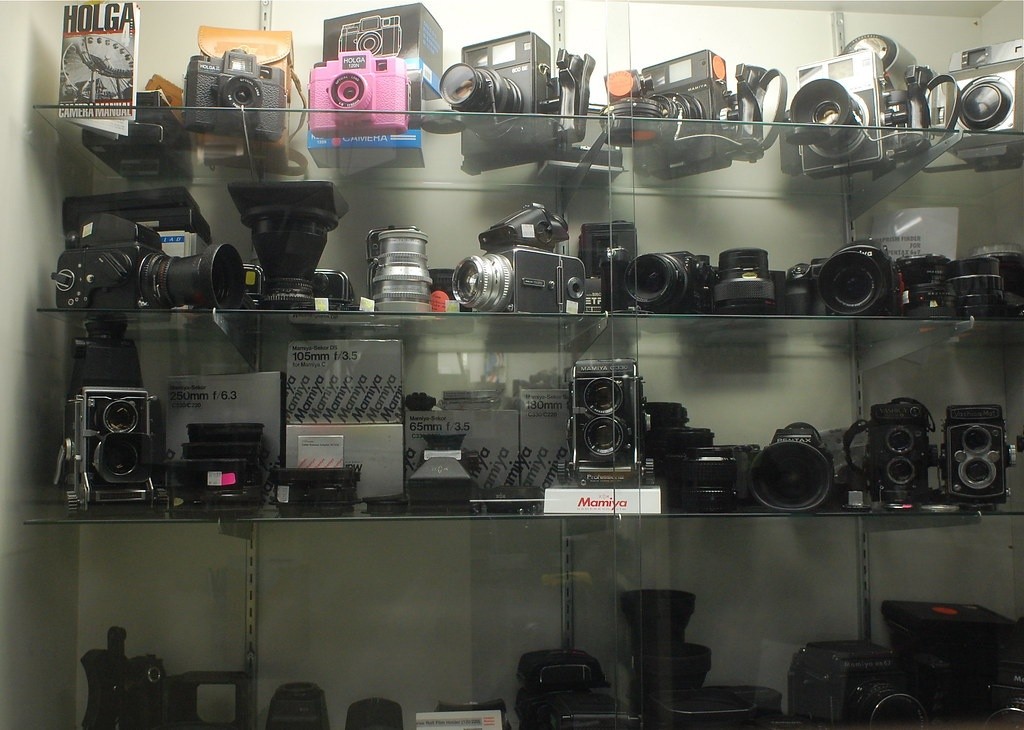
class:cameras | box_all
[51,30,1024,512]
[515,589,1024,730]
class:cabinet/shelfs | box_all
[23,103,1024,525]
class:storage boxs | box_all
[286,425,404,502]
[286,340,404,425]
[515,390,573,503]
[164,371,281,502]
[405,411,518,497]
